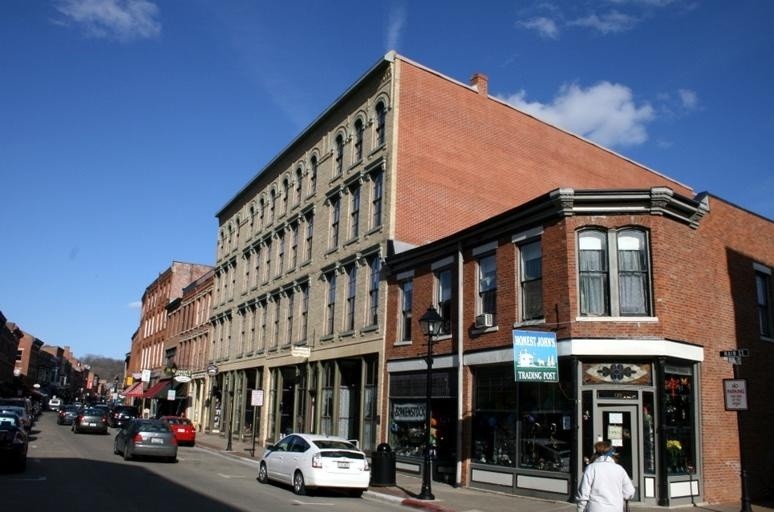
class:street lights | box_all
[165,361,177,416]
[416,304,444,500]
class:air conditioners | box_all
[475,313,493,329]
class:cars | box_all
[73,402,82,407]
[0,396,43,470]
[159,416,195,446]
[72,408,108,435]
[108,406,137,426]
[114,419,177,462]
[48,398,63,411]
[257,433,370,497]
[56,405,79,424]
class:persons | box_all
[576,440,635,512]
[429,417,438,447]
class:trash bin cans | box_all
[370,451,396,487]
